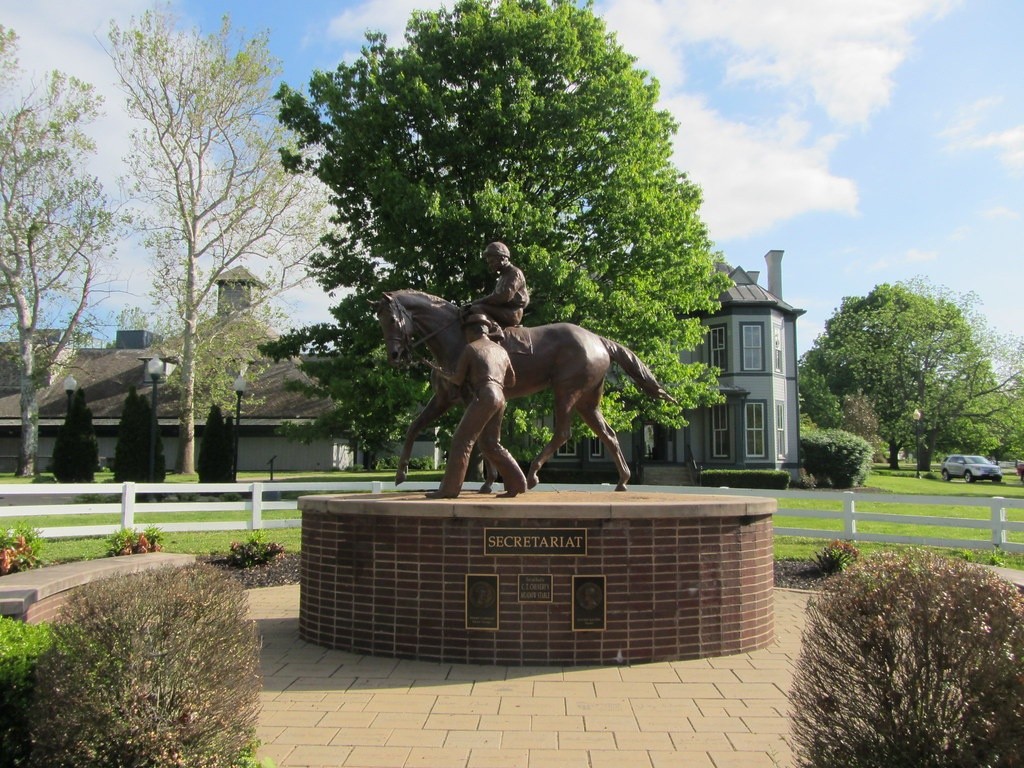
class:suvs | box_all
[942,455,1003,484]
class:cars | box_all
[1017,462,1024,483]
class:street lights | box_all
[231,375,247,483]
[147,353,165,504]
[63,373,78,412]
[912,409,921,479]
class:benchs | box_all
[0,551,196,626]
[928,562,1024,594]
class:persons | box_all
[425,313,529,498]
[460,241,530,339]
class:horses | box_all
[366,288,677,493]
